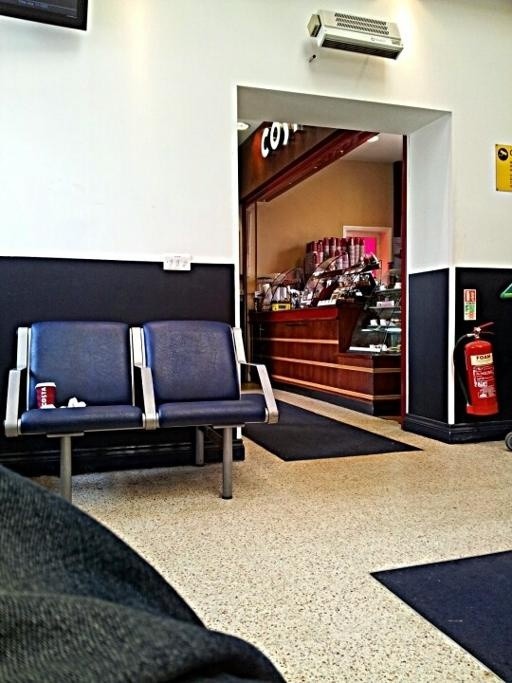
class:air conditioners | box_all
[307,9,403,60]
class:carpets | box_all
[368,549,512,683]
[242,397,425,463]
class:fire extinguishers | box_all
[453,322,500,416]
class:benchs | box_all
[4,320,279,506]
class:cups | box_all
[34,382,56,408]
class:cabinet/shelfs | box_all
[346,282,402,353]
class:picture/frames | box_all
[0,0,87,30]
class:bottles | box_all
[305,236,365,274]
